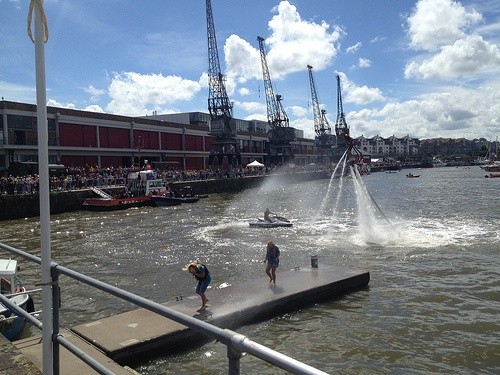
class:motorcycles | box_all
[249,212,294,228]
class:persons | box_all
[188,262,212,313]
[264,241,281,285]
[264,208,275,223]
[0,160,269,195]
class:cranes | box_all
[205,0,243,168]
[256,35,305,163]
[305,64,338,168]
[332,73,357,161]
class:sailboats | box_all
[479,136,500,172]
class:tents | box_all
[247,160,264,175]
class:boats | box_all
[151,194,199,205]
[82,195,153,209]
[485,173,500,178]
[406,172,419,177]
